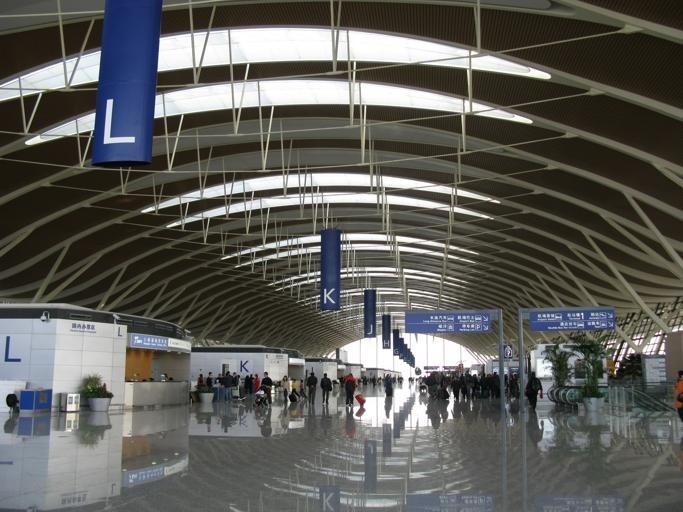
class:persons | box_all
[291,378,300,399]
[408,368,519,399]
[218,394,394,439]
[339,372,404,407]
[306,372,318,405]
[168,377,173,380]
[281,376,290,402]
[300,379,307,398]
[321,373,331,406]
[417,392,545,449]
[526,371,543,410]
[197,371,273,410]
[164,374,168,380]
[673,370,683,421]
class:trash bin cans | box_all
[60,393,80,412]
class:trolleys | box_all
[231,384,248,402]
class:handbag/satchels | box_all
[329,386,332,390]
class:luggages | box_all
[288,391,297,402]
[354,390,366,404]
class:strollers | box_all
[253,385,270,409]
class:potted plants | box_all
[566,330,606,412]
[82,374,115,413]
[195,385,216,403]
